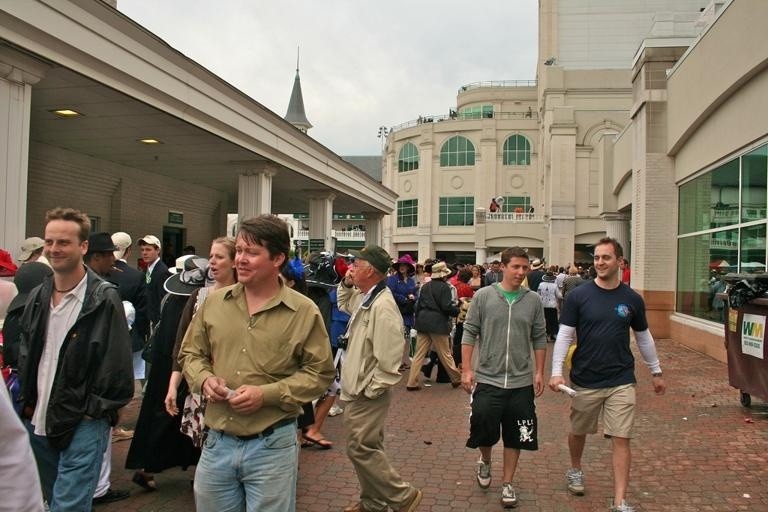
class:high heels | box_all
[132,472,156,490]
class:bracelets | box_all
[652,373,663,377]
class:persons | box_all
[489,198,500,212]
[336,245,423,511]
[164,236,240,486]
[179,211,337,512]
[123,255,211,491]
[527,206,534,212]
[460,245,547,506]
[18,208,134,510]
[1,370,46,511]
[547,236,666,512]
[1,233,637,450]
[703,269,728,321]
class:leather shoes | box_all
[92,488,130,504]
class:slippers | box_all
[301,433,331,448]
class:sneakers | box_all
[393,489,423,512]
[342,501,361,512]
[564,468,585,495]
[475,454,492,489]
[112,427,135,443]
[398,363,461,392]
[501,481,518,508]
[608,498,636,512]
[328,403,344,417]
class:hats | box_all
[302,244,453,288]
[531,258,557,282]
[1,232,216,315]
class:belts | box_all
[236,417,296,441]
